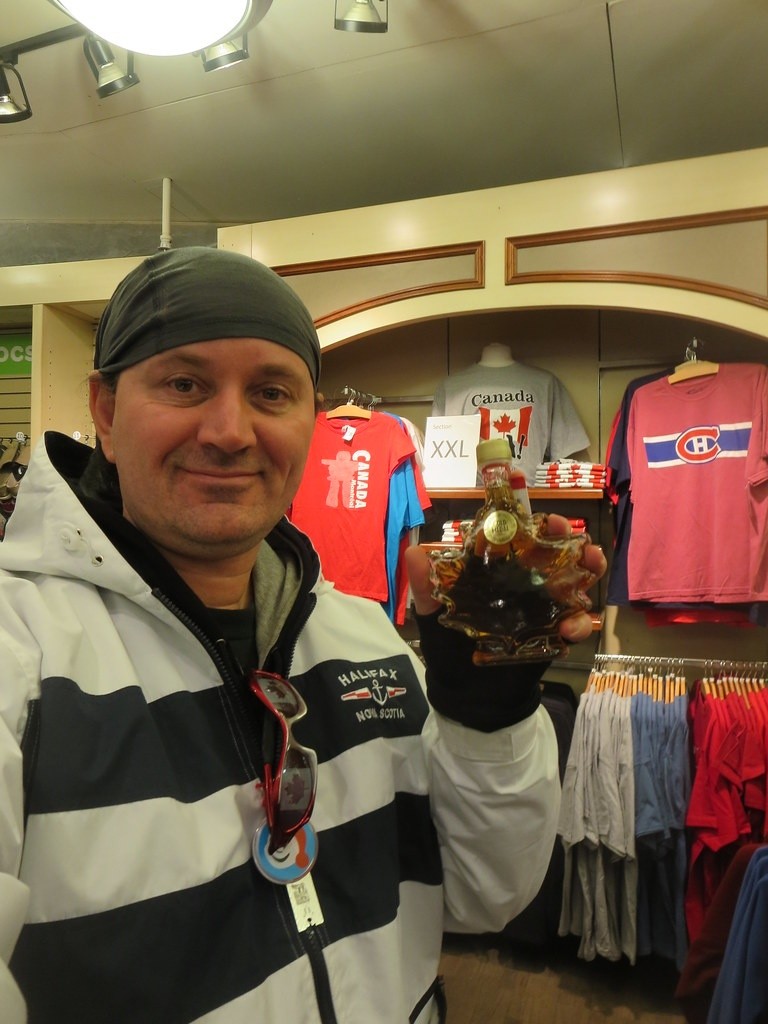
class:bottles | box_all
[424,438,589,667]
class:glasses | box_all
[245,667,317,856]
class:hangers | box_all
[583,653,768,711]
[323,385,378,422]
[665,335,720,384]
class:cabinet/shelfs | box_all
[411,482,613,636]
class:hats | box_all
[93,246,322,391]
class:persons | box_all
[0,246,606,1024]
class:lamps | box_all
[334,0,390,34]
[48,0,276,59]
[82,32,141,100]
[0,62,33,125]
[200,32,250,74]
